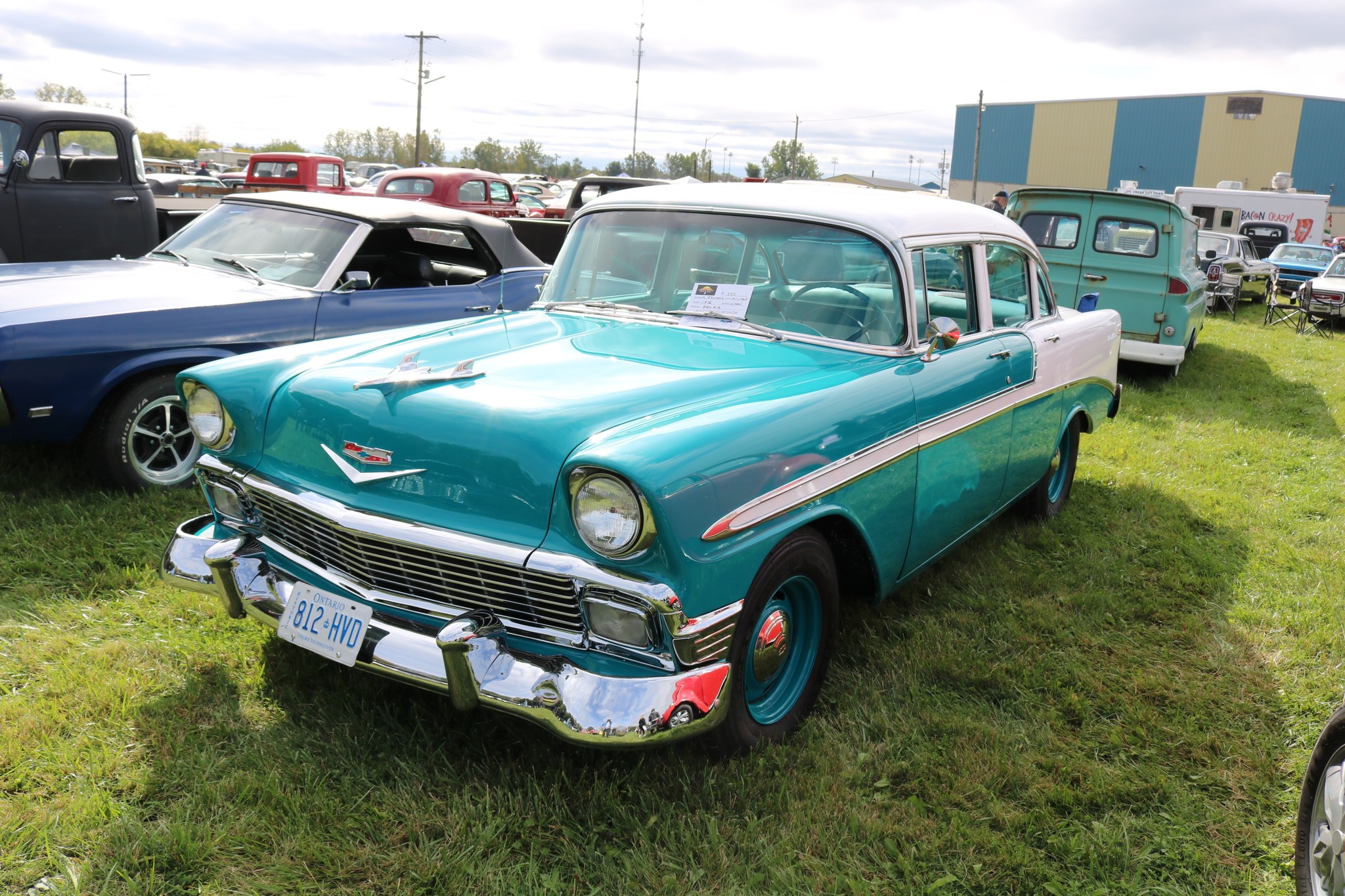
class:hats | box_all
[201,163,206,166]
[994,191,1006,197]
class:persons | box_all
[194,162,211,177]
[522,175,558,184]
[1322,240,1345,255]
[1096,221,1114,252]
[980,191,1007,214]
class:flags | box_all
[1328,214,1332,227]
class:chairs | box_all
[1248,230,1255,235]
[1270,231,1279,237]
[258,170,272,177]
[1319,253,1331,263]
[375,251,435,288]
[459,189,470,200]
[1296,250,1313,259]
[1205,274,1243,321]
[284,170,297,178]
[1264,267,1345,340]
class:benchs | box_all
[743,299,931,345]
[32,155,122,182]
